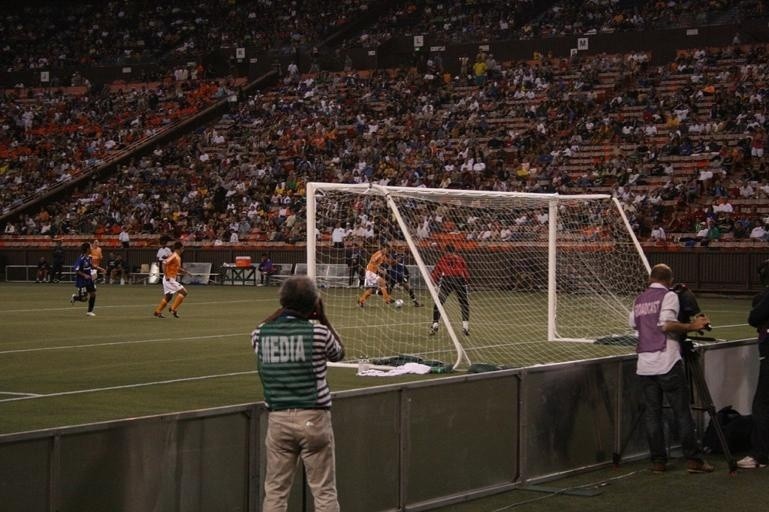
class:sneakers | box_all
[386,299,395,304]
[428,325,438,336]
[415,303,425,307]
[647,461,666,474]
[169,307,180,319]
[737,455,767,469]
[357,300,364,307]
[86,312,95,316]
[70,293,75,305]
[462,328,471,336]
[154,311,165,318]
[687,458,715,473]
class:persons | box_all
[90,240,103,290]
[154,241,191,318]
[347,242,363,287]
[629,263,715,473]
[71,243,96,316]
[430,242,471,336]
[357,243,396,308]
[155,238,172,283]
[99,252,117,284]
[36,256,49,282]
[252,276,344,512]
[256,252,272,286]
[388,246,426,307]
[111,255,130,285]
[1,0,766,249]
[747,295,768,328]
[733,260,769,469]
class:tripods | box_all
[613,341,737,477]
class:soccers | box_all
[396,300,403,307]
